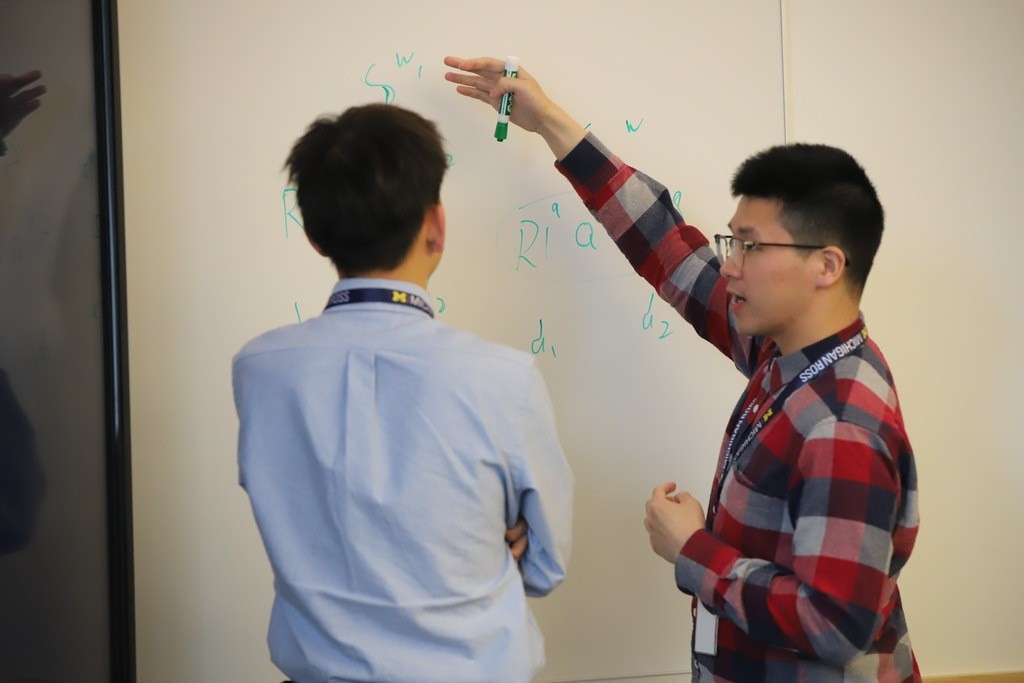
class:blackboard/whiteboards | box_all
[92,0,1024,681]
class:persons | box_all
[231,103,574,683]
[444,56,921,681]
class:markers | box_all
[493,56,518,142]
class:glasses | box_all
[714,234,851,270]
